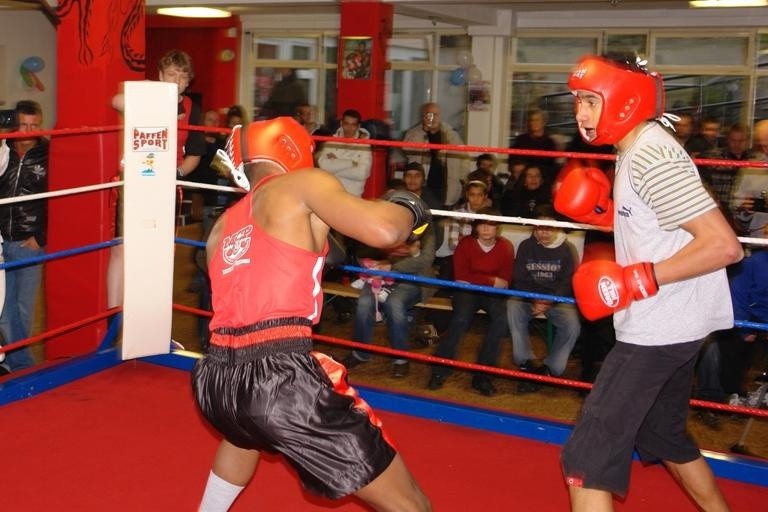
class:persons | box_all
[346,43,370,79]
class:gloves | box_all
[381,189,433,239]
[553,167,615,227]
[572,260,658,321]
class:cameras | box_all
[747,197,768,213]
[0,109,19,128]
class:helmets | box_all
[210,117,316,193]
[568,58,665,146]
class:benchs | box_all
[318,276,557,357]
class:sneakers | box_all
[727,372,768,410]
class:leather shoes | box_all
[472,375,497,396]
[340,354,364,371]
[392,361,409,378]
[429,374,448,390]
[517,358,550,393]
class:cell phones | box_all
[427,112,433,127]
